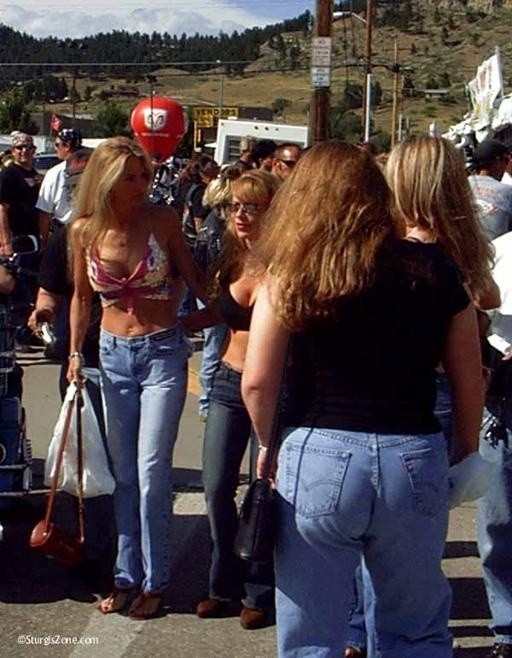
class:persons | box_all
[0,124,512,657]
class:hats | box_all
[251,140,276,158]
[65,148,92,185]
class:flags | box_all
[51,112,64,131]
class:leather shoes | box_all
[241,605,274,628]
[196,598,229,616]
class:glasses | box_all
[276,159,296,169]
[227,202,261,212]
[14,145,32,151]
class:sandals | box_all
[99,587,165,620]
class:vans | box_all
[99,83,139,98]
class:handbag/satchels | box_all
[182,200,199,238]
[232,475,278,566]
[30,518,84,567]
[45,284,71,360]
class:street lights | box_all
[332,11,398,150]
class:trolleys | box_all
[0,306,81,548]
